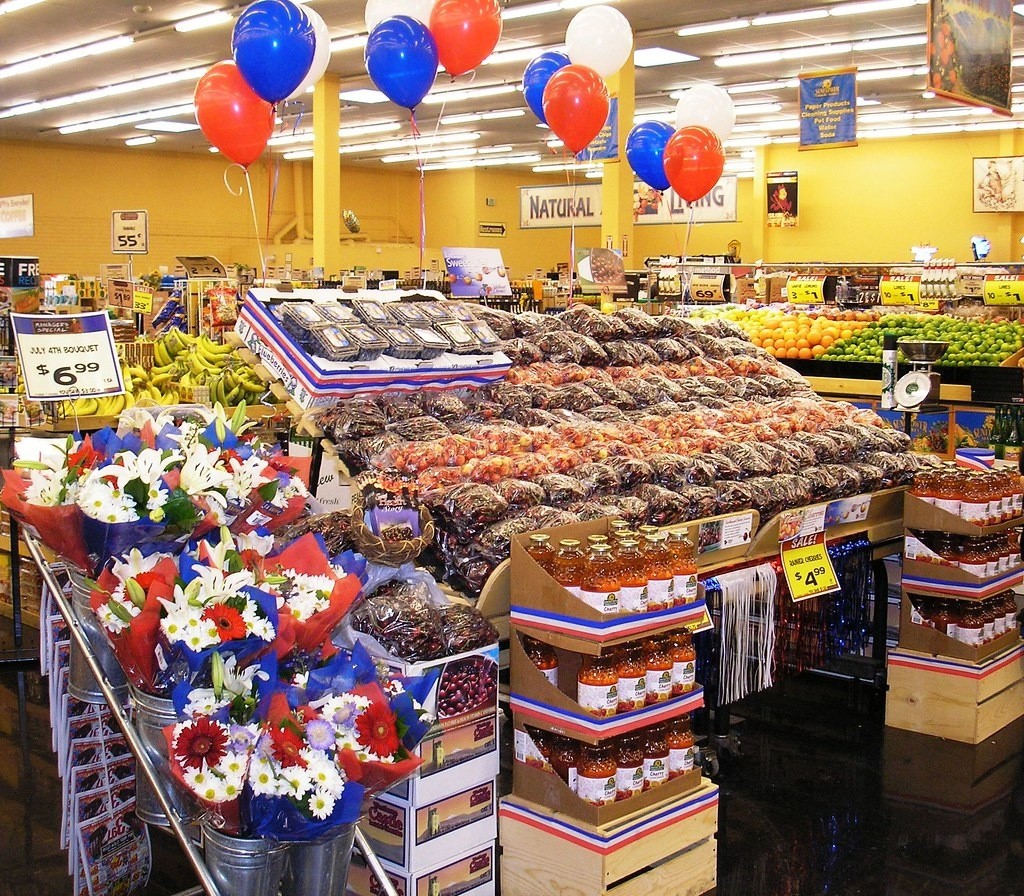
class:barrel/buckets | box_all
[61,564,359,896]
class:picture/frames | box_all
[972,155,1024,214]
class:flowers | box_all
[15,420,432,829]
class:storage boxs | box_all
[897,580,1023,663]
[902,487,1024,537]
[334,642,500,734]
[359,775,498,875]
[370,710,500,807]
[512,709,705,827]
[348,839,496,896]
[232,285,514,413]
[899,525,1024,586]
[507,618,709,723]
[506,515,708,622]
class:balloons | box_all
[662,127,725,205]
[193,59,275,175]
[542,64,610,157]
[674,82,735,143]
[625,120,678,194]
[283,4,332,101]
[230,0,316,103]
[564,5,633,80]
[522,51,573,129]
[364,0,437,37]
[429,1,503,77]
[364,16,438,111]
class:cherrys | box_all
[475,303,744,340]
[348,580,500,661]
[314,336,935,596]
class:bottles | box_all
[478,288,565,316]
[523,626,696,806]
[909,406,1024,648]
[526,519,698,611]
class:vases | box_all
[285,825,356,896]
[131,686,203,827]
[203,815,283,896]
[67,565,131,705]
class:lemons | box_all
[691,308,806,330]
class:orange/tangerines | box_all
[813,316,1024,368]
[746,315,875,360]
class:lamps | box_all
[0,0,1024,181]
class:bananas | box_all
[11,328,269,417]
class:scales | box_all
[893,339,951,412]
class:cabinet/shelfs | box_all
[885,570,1024,748]
[496,602,719,896]
[14,342,290,631]
